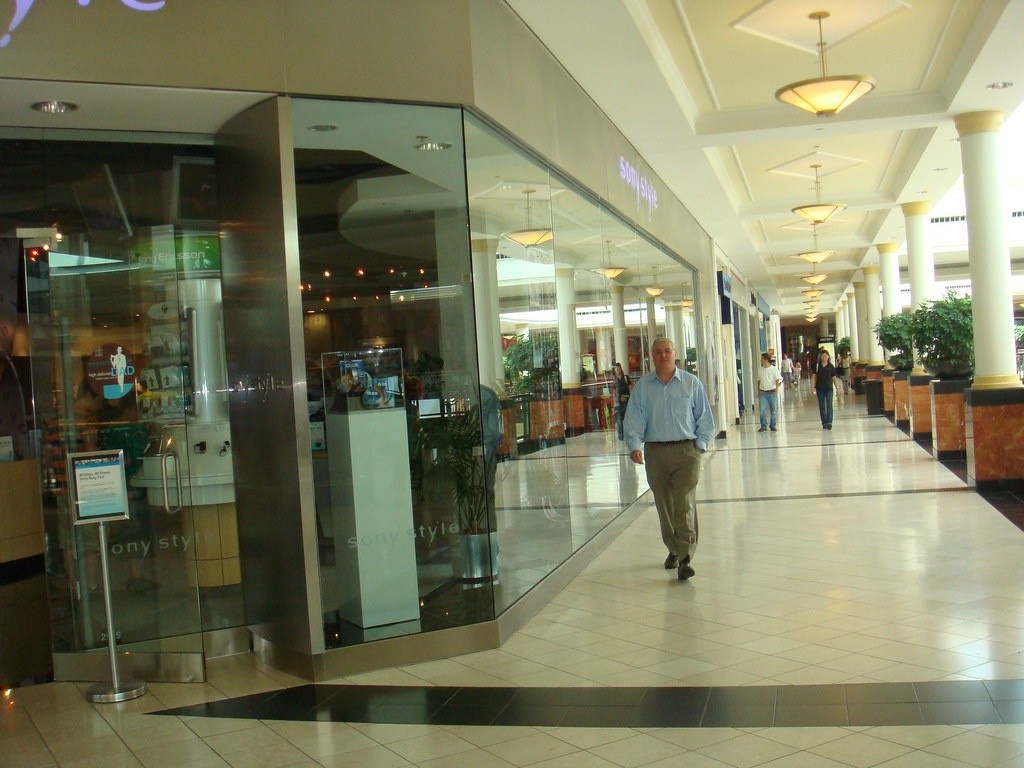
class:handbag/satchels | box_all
[620,395,629,402]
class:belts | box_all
[645,440,689,444]
[760,390,774,392]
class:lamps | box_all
[644,267,664,296]
[673,283,693,307]
[790,165,848,225]
[801,263,828,285]
[800,284,824,323]
[798,235,836,265]
[588,241,626,279]
[774,12,875,118]
[500,191,553,249]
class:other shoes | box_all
[664,553,679,569]
[823,424,832,430]
[618,436,624,441]
[758,428,767,432]
[771,427,777,431]
[678,563,695,581]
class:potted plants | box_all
[871,310,913,373]
[904,290,975,381]
[411,374,522,579]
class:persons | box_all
[781,354,794,390]
[756,353,780,433]
[398,360,424,439]
[615,363,633,440]
[813,350,840,430]
[839,349,854,394]
[793,362,801,387]
[44,379,161,595]
[623,337,714,579]
[817,347,831,363]
[342,369,365,393]
